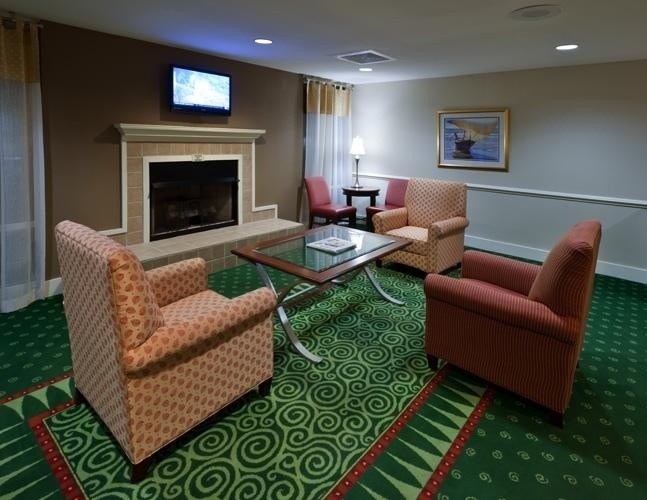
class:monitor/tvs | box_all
[170,64,233,116]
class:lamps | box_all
[350,136,366,188]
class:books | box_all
[305,236,355,254]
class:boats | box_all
[453,131,476,151]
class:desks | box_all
[230,224,406,363]
[342,187,379,227]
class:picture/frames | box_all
[436,106,511,172]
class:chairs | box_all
[424,220,603,428]
[54,219,278,485]
[366,179,408,225]
[304,178,357,229]
[371,182,470,274]
